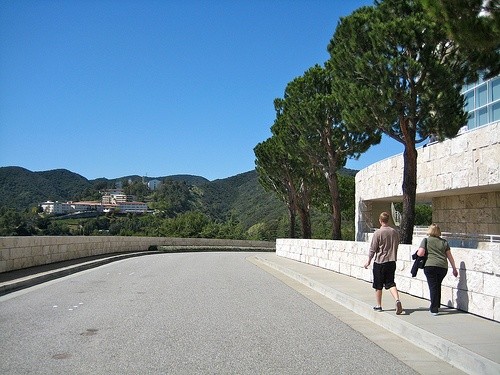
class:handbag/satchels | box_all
[415,238,429,268]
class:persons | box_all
[416,223,458,317]
[364,212,403,315]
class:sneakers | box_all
[373,305,382,312]
[395,300,402,315]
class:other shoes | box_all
[430,309,440,316]
